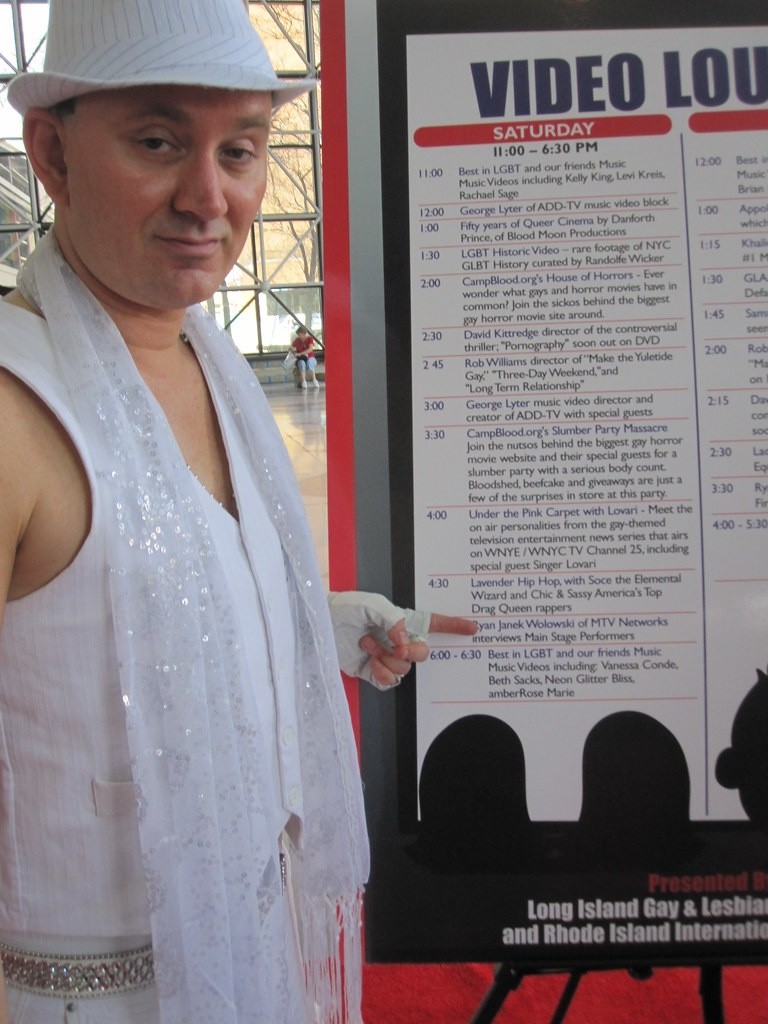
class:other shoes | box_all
[301,379,307,388]
[312,379,320,388]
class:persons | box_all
[289,328,320,388]
[0,0,477,1024]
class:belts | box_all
[0,950,151,998]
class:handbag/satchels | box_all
[282,351,297,371]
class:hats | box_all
[7,0,317,117]
